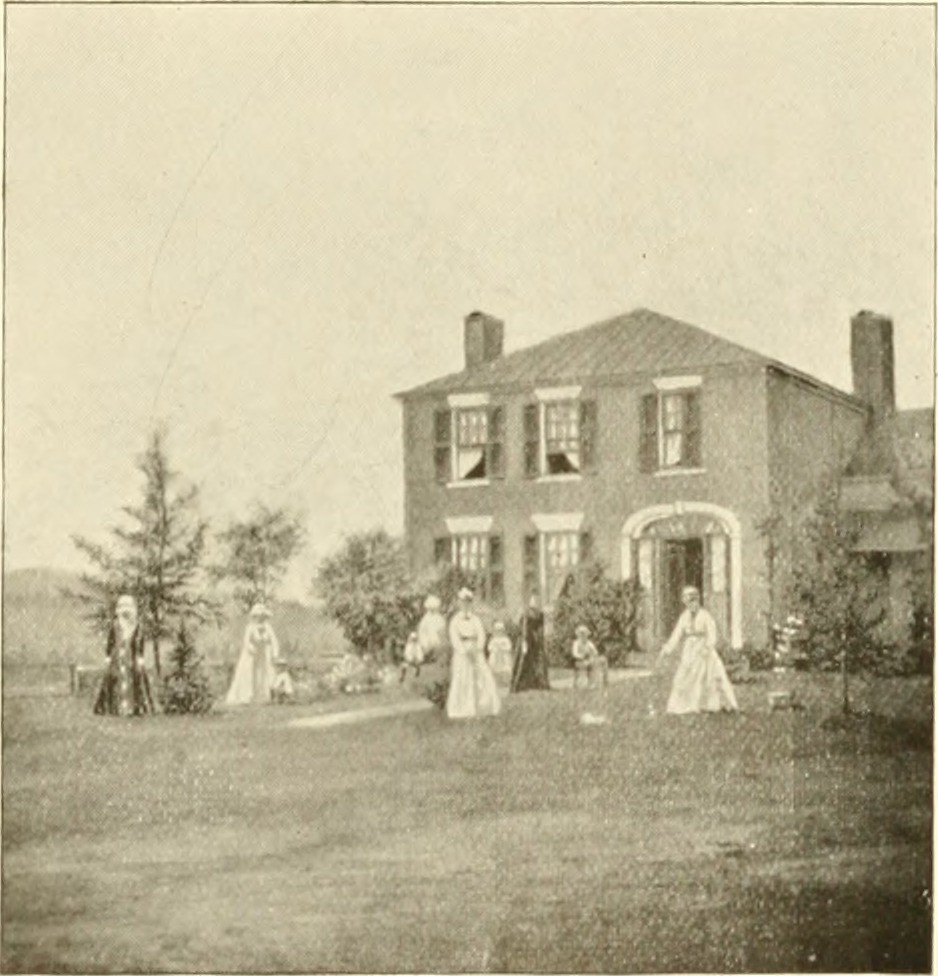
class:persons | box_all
[222,602,280,706]
[94,594,162,717]
[657,587,738,713]
[398,585,608,716]
